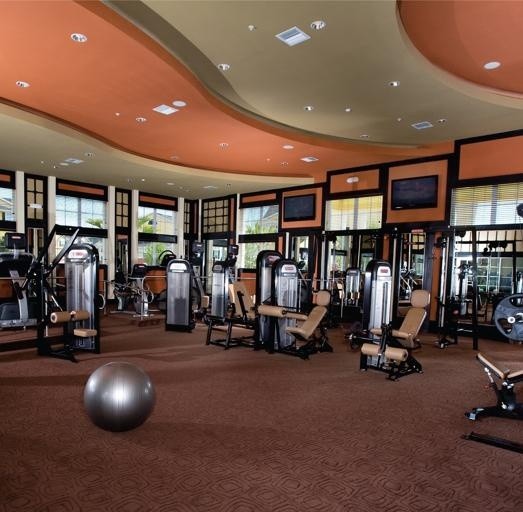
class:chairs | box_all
[284,290,333,360]
[370,289,431,381]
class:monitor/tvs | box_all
[391,174,438,210]
[284,194,315,221]
